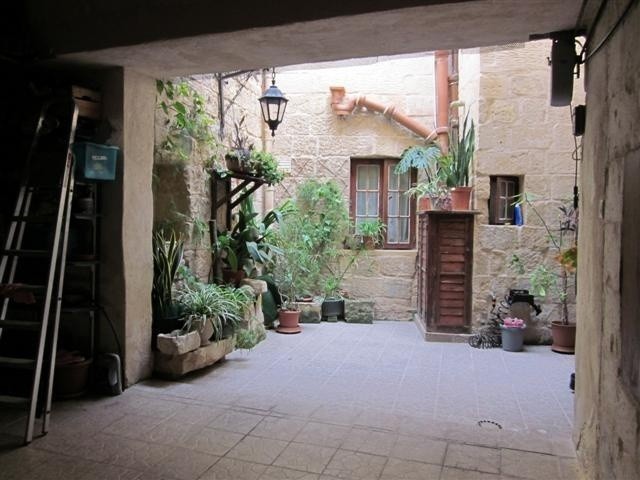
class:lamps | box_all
[216,65,289,138]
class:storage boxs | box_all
[73,141,118,182]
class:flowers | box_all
[502,317,525,328]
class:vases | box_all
[56,357,95,400]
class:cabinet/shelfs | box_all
[416,211,474,333]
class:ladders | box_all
[0,96,80,444]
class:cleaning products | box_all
[515,203,523,225]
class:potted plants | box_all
[273,179,345,334]
[225,145,291,188]
[153,209,270,346]
[511,192,575,353]
[359,218,388,251]
[394,108,477,211]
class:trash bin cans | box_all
[510,289,534,345]
